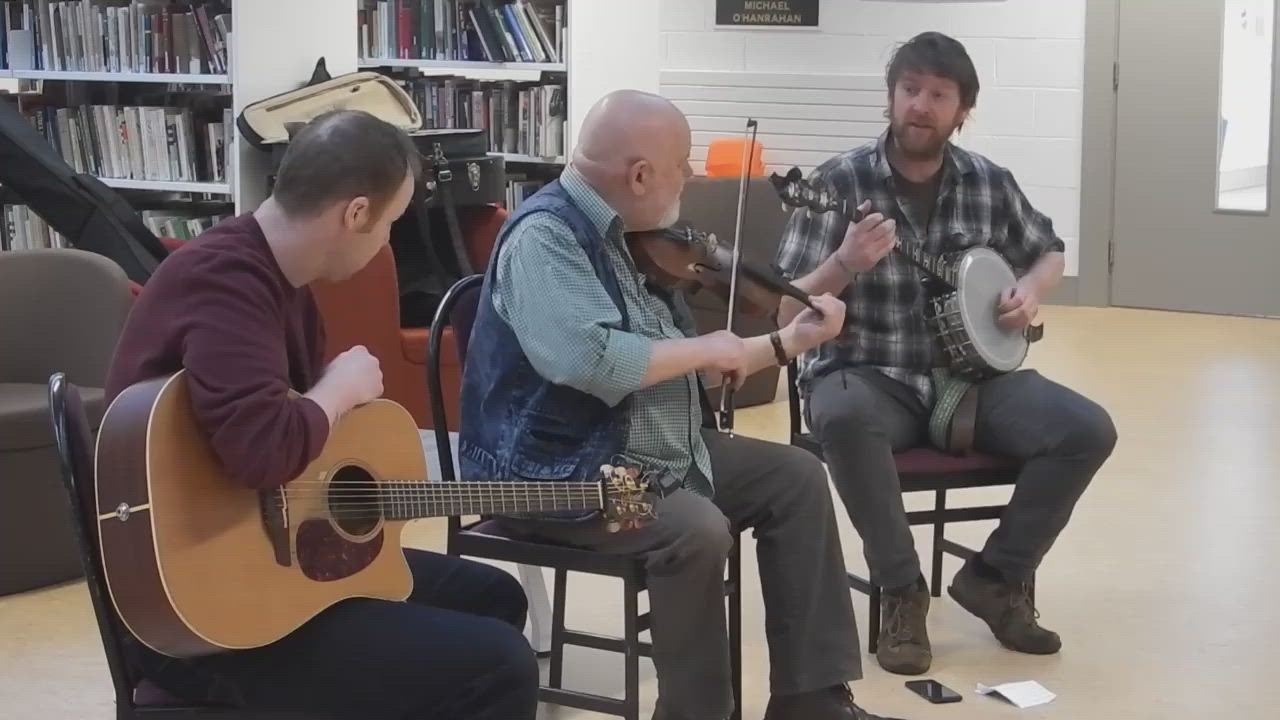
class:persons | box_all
[773,27,1118,676]
[88,110,540,720]
[456,86,904,720]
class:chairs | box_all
[427,274,743,720]
[48,373,247,720]
[770,311,1035,655]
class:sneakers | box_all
[947,562,1063,653]
[876,573,933,673]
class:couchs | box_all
[310,209,508,432]
[678,176,794,411]
[0,249,132,597]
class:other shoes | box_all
[763,683,909,720]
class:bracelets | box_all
[769,330,788,367]
[830,250,860,283]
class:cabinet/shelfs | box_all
[356,0,573,165]
[0,0,268,218]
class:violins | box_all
[624,226,820,317]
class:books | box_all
[0,0,569,290]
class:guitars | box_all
[767,165,1047,380]
[92,365,662,658]
